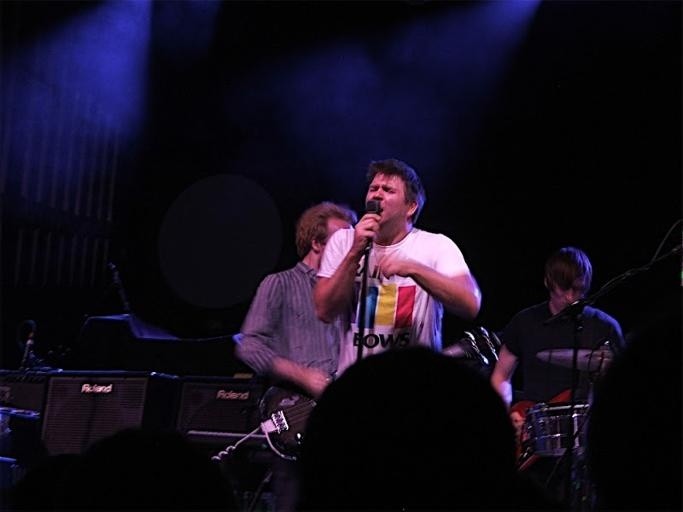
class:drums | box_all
[527,401,591,459]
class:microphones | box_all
[363,199,380,252]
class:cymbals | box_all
[540,346,608,372]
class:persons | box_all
[1,429,229,511]
[491,249,625,508]
[233,201,357,510]
[300,345,549,512]
[312,158,482,371]
[593,308,680,512]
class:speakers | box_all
[39,377,149,457]
[177,381,268,458]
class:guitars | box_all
[257,326,503,460]
